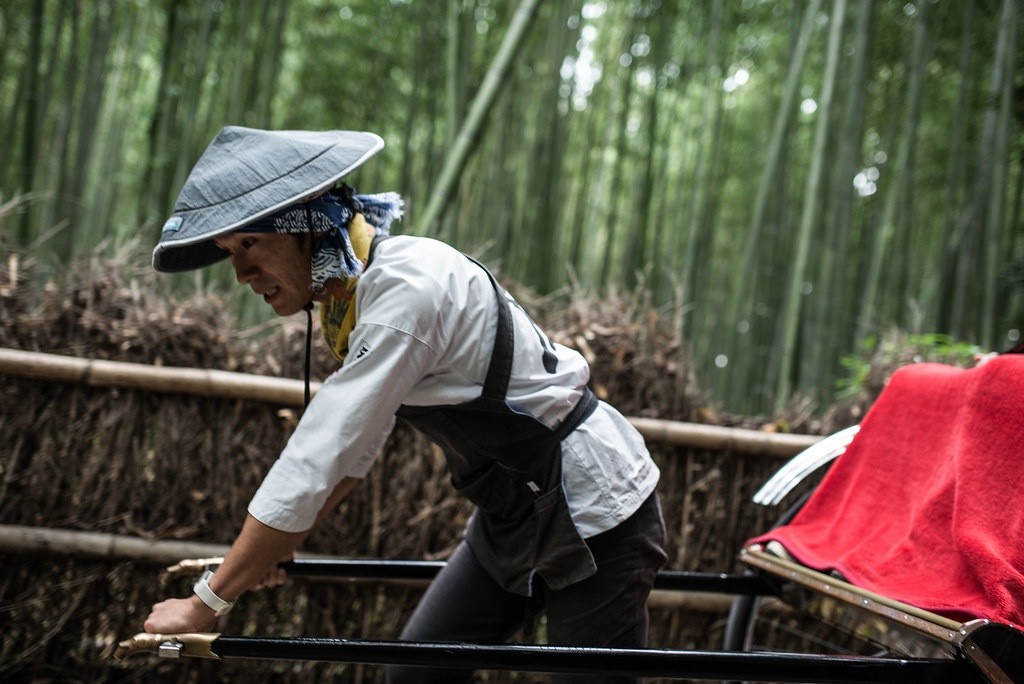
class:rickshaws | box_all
[115,352,1023,683]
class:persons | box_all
[138,115,674,681]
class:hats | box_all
[151,125,382,273]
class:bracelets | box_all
[194,571,238,618]
